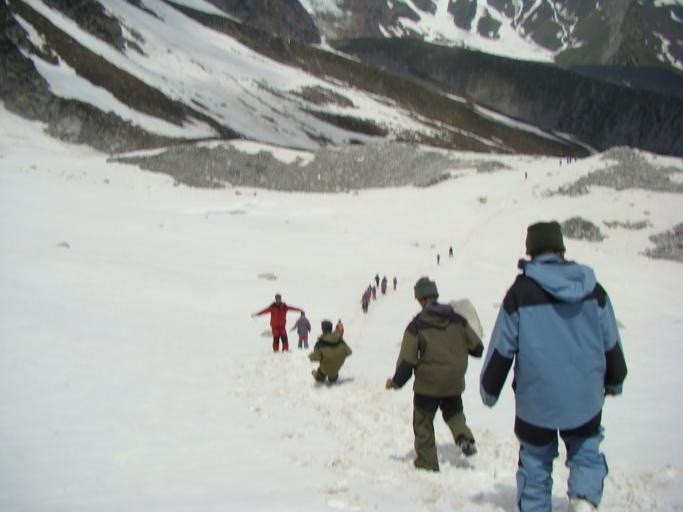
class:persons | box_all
[361,273,398,315]
[292,311,312,349]
[307,319,353,382]
[385,275,484,473]
[336,319,344,340]
[252,293,302,351]
[479,220,628,512]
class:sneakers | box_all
[414,459,439,469]
[312,370,322,382]
[458,436,476,455]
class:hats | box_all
[322,322,332,330]
[415,278,439,299]
[526,222,565,256]
